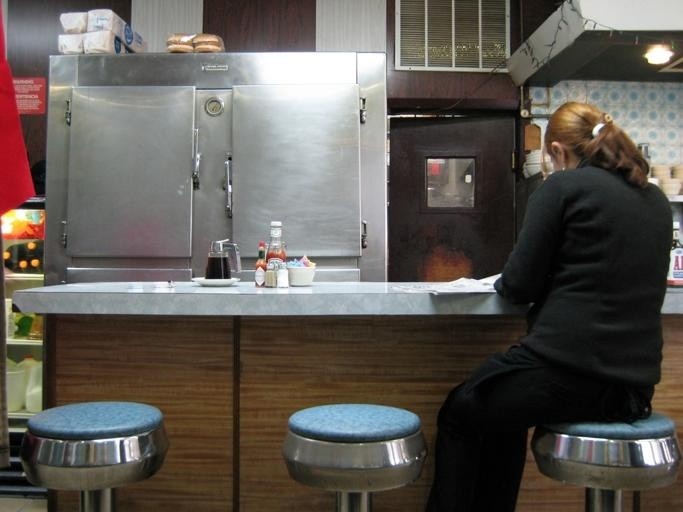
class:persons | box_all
[418,99,674,512]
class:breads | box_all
[166,34,224,53]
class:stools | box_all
[19,401,170,512]
[530,413,683,512]
[280,402,429,512]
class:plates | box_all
[191,277,240,286]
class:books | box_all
[393,268,503,298]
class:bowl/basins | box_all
[651,164,683,195]
[525,149,551,177]
[285,267,316,287]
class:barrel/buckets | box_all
[6,370,25,413]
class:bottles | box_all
[667,221,683,288]
[205,239,241,279]
[4,240,43,272]
[254,221,289,288]
